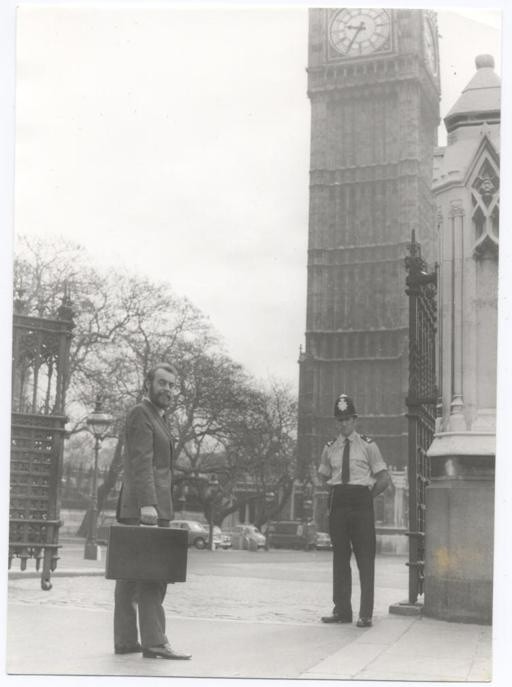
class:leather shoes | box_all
[322,615,352,623]
[357,618,372,627]
[115,643,192,660]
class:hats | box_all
[334,394,356,417]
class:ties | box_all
[342,440,350,485]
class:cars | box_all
[166,518,333,549]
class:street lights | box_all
[84,408,113,559]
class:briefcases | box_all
[105,519,189,582]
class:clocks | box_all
[327,8,390,58]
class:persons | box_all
[316,393,392,628]
[106,362,194,661]
